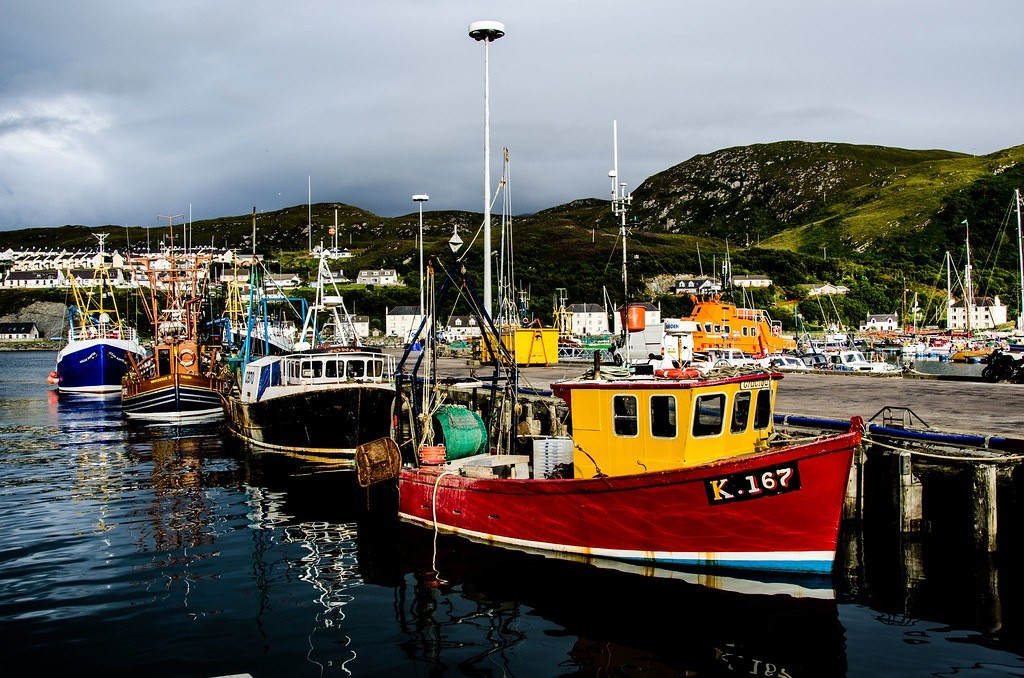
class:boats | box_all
[395,121,868,580]
[48,171,481,487]
[357,505,850,678]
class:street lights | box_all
[469,20,506,332]
[413,192,422,311]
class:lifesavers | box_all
[89,327,97,339]
[654,368,702,379]
[908,326,914,332]
[934,338,944,347]
[874,354,883,362]
[178,349,196,367]
[822,346,848,352]
[109,330,121,340]
[773,325,781,336]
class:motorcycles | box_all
[981,345,1024,384]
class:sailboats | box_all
[514,118,1024,371]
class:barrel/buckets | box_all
[619,306,647,331]
[419,443,447,473]
